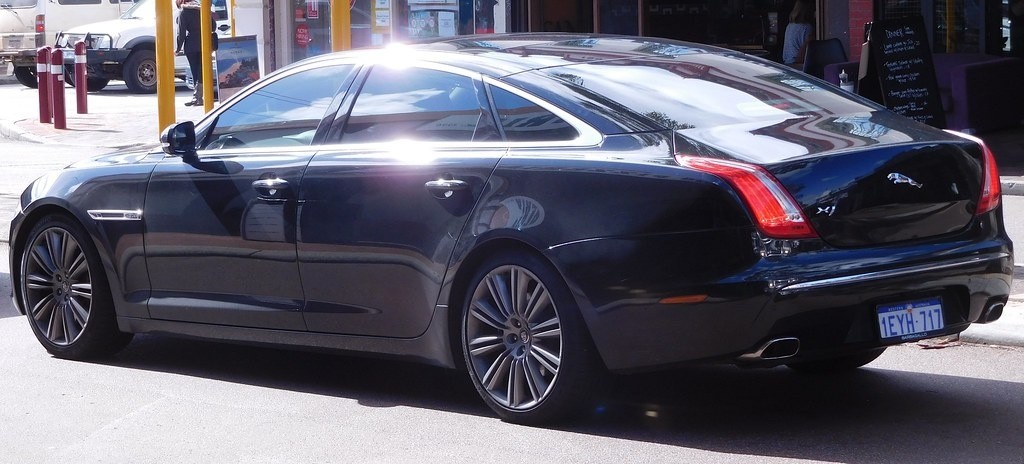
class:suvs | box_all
[52,0,262,96]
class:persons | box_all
[783,0,816,65]
[175,0,216,107]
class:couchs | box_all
[824,53,1024,133]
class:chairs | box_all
[803,38,846,73]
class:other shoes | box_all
[916,332,964,349]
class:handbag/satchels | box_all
[212,32,219,51]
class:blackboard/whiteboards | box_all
[858,15,948,130]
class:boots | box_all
[184,83,203,106]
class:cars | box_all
[9,29,1015,425]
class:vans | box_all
[0,0,138,89]
